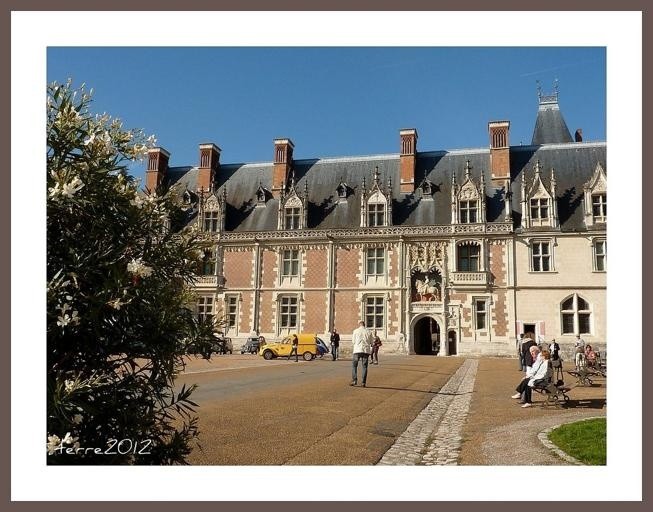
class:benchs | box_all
[527,382,569,409]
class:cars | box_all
[188,332,330,361]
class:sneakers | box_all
[511,393,532,408]
[349,379,365,387]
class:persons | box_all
[260,339,266,345]
[369,330,382,365]
[287,334,298,362]
[220,335,225,352]
[575,129,582,142]
[349,321,373,387]
[421,275,429,293]
[433,341,436,350]
[511,332,597,408]
[330,329,340,361]
